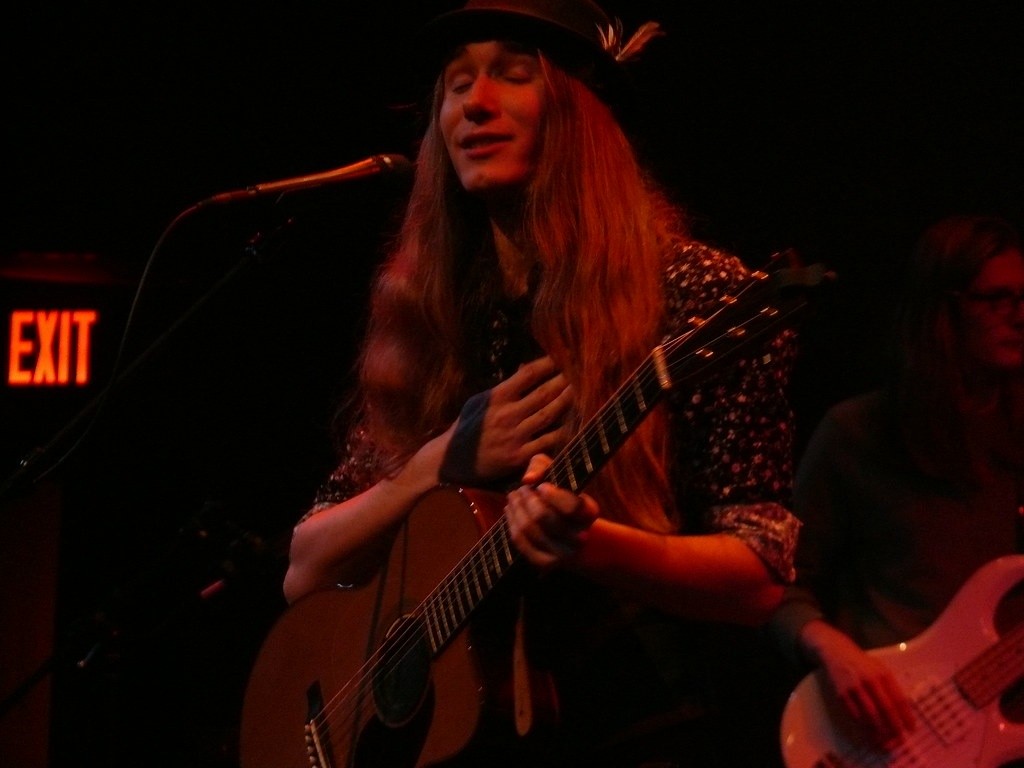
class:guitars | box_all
[243,257,799,768]
[781,556,1024,767]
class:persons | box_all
[770,214,1024,753]
[283,0,801,768]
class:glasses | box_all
[960,285,1024,316]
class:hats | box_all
[414,0,641,106]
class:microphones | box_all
[211,154,414,207]
